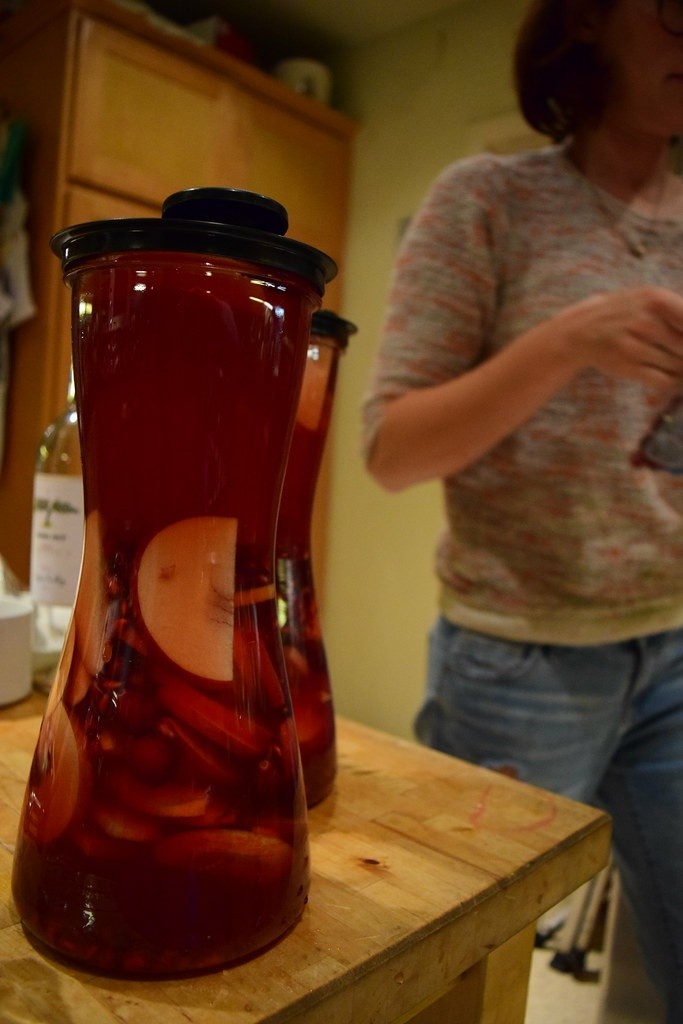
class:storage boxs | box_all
[188,15,251,63]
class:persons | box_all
[362,0,683,1024]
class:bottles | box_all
[31,358,95,698]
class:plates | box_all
[34,603,82,672]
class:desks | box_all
[0,687,611,1024]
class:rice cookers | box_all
[279,58,333,108]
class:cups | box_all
[10,184,357,982]
[1,596,35,707]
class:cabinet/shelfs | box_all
[0,0,358,617]
